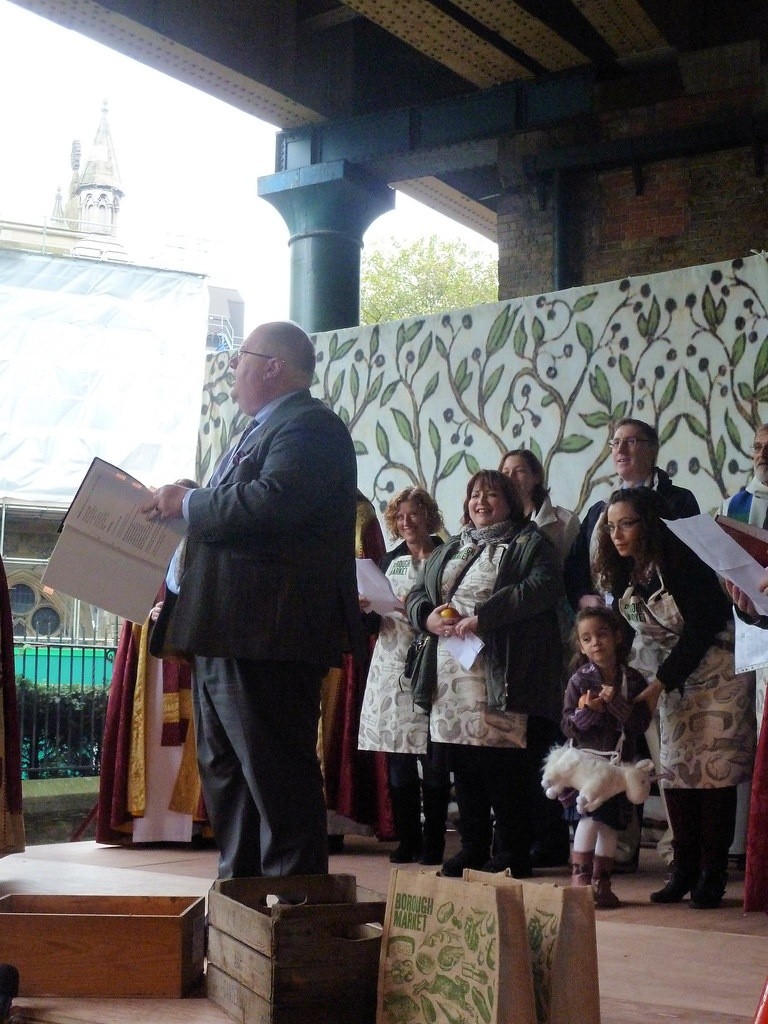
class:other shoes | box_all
[441,841,492,877]
[481,852,533,878]
[529,846,568,867]
[616,849,640,873]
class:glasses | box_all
[601,518,642,534]
[608,436,652,448]
[750,443,768,456]
[235,345,288,369]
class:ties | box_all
[222,420,260,476]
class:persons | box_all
[147,321,365,958]
[96,419,768,913]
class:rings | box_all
[443,630,449,637]
[153,506,162,514]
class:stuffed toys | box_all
[540,740,675,816]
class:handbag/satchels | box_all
[376,867,536,1024]
[403,631,438,709]
[464,868,600,1024]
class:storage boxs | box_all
[0,894,206,1000]
[207,873,387,1024]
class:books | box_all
[42,456,187,628]
[714,514,768,569]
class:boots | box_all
[650,843,700,903]
[389,780,422,863]
[688,845,728,908]
[570,849,595,886]
[590,857,620,908]
[418,781,450,865]
